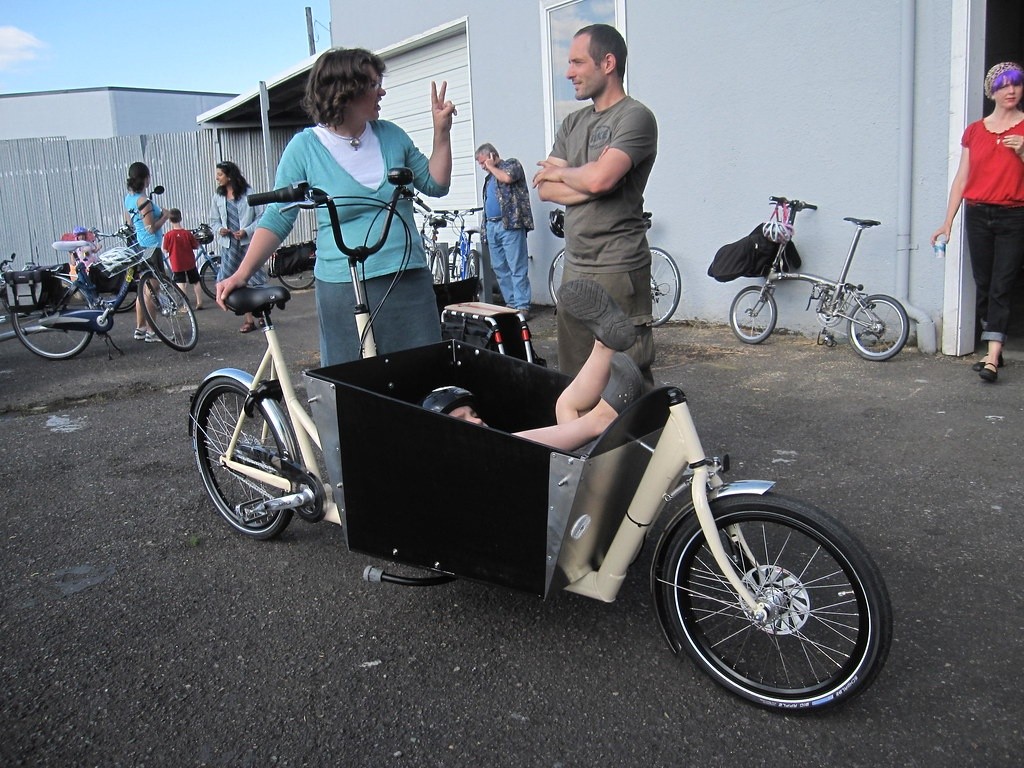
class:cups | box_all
[934,240,945,259]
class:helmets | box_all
[549,208,564,238]
[763,221,793,243]
[422,385,487,419]
[73,227,86,235]
[194,230,214,243]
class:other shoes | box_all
[972,353,1004,370]
[979,363,998,381]
[518,309,530,321]
[196,305,202,310]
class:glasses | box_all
[371,82,382,92]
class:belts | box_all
[487,218,501,222]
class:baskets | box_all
[91,224,159,278]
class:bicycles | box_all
[727,195,911,360]
[2,197,199,361]
[434,206,486,285]
[267,227,318,289]
[409,207,451,285]
[94,223,225,313]
[546,208,683,327]
[184,165,891,720]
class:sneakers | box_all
[133,329,147,339]
[145,331,174,342]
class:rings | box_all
[1015,142,1017,145]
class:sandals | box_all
[599,351,644,415]
[240,321,257,333]
[557,280,637,351]
[259,318,265,327]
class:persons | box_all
[475,143,534,322]
[216,47,457,367]
[123,162,171,342]
[210,161,266,333]
[422,279,645,452]
[533,24,657,395]
[932,61,1024,383]
[163,208,205,310]
[69,226,102,301]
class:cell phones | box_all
[489,152,498,163]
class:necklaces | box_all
[315,121,369,152]
